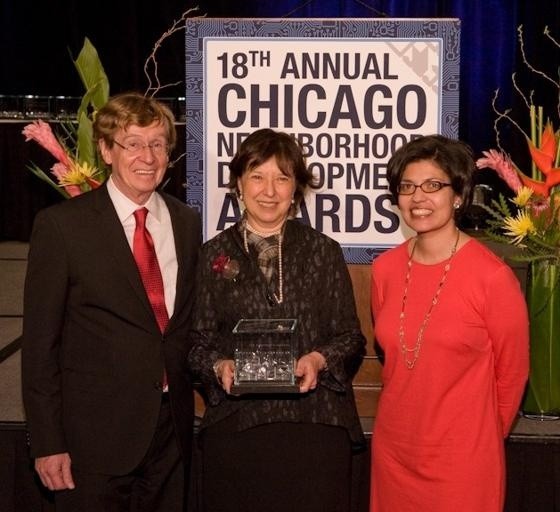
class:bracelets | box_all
[213,359,223,387]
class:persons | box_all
[370,135,530,512]
[189,127,367,512]
[21,94,202,512]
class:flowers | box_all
[22,36,112,200]
[473,25,560,414]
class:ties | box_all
[132,207,170,389]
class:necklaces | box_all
[399,227,461,370]
[242,220,286,310]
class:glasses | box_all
[111,138,168,152]
[396,181,452,195]
